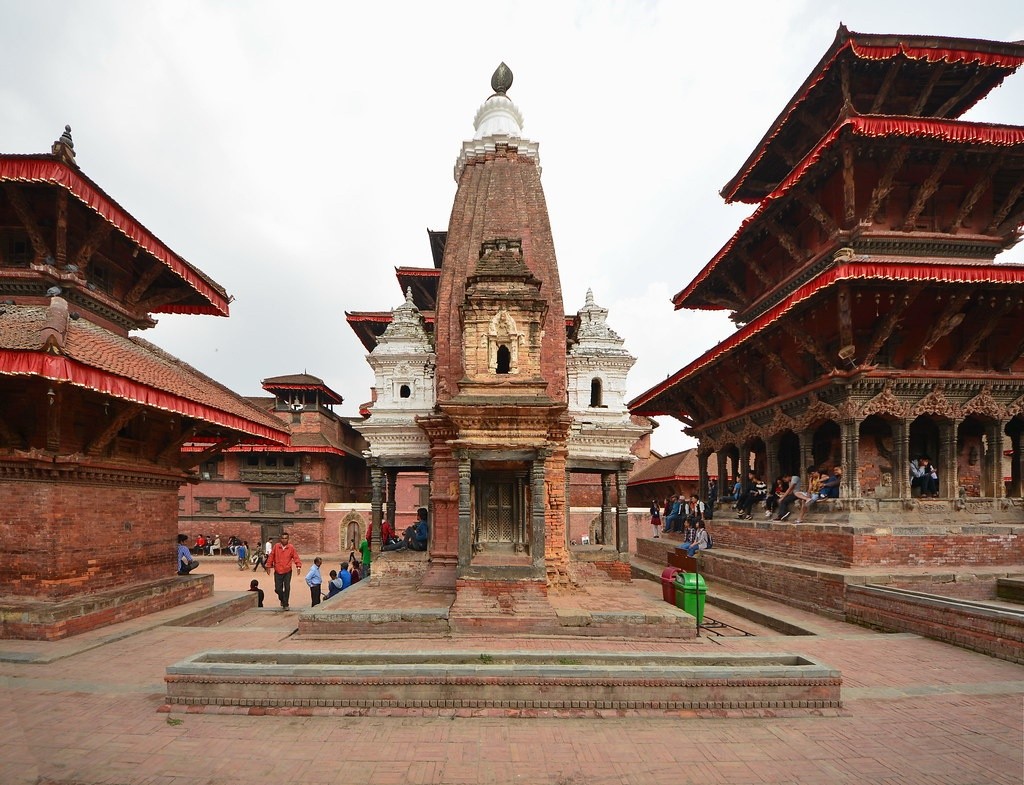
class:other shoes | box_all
[793,520,804,523]
[744,515,752,520]
[737,511,745,515]
[731,505,738,509]
[920,495,927,498]
[771,515,783,522]
[805,498,812,504]
[765,510,772,516]
[653,535,659,538]
[283,606,289,611]
[932,495,938,498]
[781,512,791,520]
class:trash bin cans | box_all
[672,571,709,624]
[660,565,687,606]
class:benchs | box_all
[189,546,227,556]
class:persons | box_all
[304,557,323,607]
[359,541,371,578]
[595,530,599,544]
[660,494,705,533]
[265,533,302,611]
[247,579,264,607]
[193,533,241,556]
[708,479,717,508]
[650,499,661,538]
[236,538,273,572]
[346,539,357,562]
[366,511,396,550]
[718,464,842,523]
[401,508,428,552]
[497,313,510,336]
[178,534,199,575]
[323,561,363,600]
[679,520,708,556]
[909,455,939,498]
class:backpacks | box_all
[698,500,713,520]
[700,530,713,548]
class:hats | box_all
[216,534,219,537]
[671,494,678,499]
[679,496,685,500]
[339,561,348,568]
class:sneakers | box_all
[816,494,828,502]
